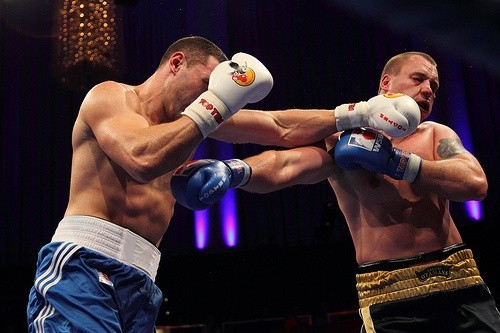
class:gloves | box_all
[170,158,249,212]
[335,93,422,137]
[334,127,422,185]
[178,51,274,140]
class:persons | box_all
[26,36,420,333]
[170,52,500,333]
